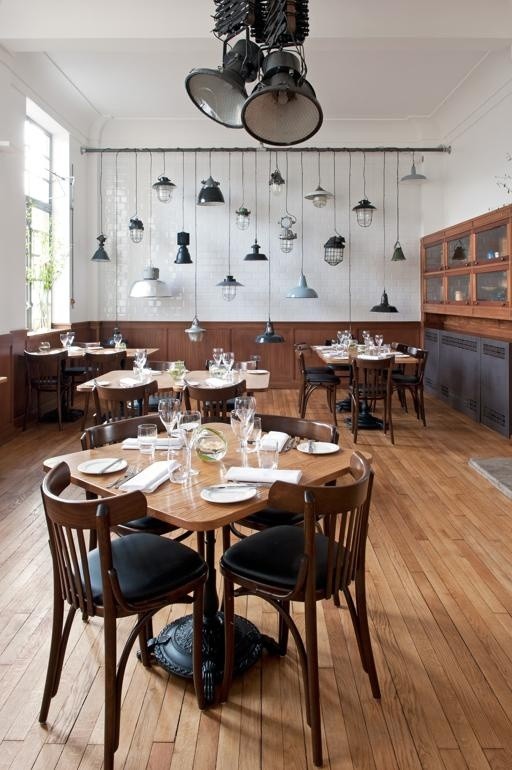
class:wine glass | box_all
[134,348,234,381]
[59,334,67,351]
[336,329,383,357]
[114,334,122,352]
[67,332,76,347]
[158,397,256,483]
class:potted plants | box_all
[38,215,65,329]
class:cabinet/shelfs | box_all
[419,204,512,440]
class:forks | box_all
[104,464,143,489]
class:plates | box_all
[77,458,127,475]
[247,370,268,374]
[88,347,103,349]
[394,355,410,358]
[200,484,257,504]
[358,355,378,360]
[205,378,224,387]
[297,442,341,454]
[84,381,109,387]
[104,351,115,354]
[481,287,497,291]
[120,378,141,385]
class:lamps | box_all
[184,1,323,146]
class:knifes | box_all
[284,438,295,452]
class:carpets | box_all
[468,456,511,501]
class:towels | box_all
[118,460,183,492]
[224,465,301,485]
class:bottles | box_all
[115,343,126,352]
[455,280,463,302]
[38,342,50,353]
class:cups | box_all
[137,423,157,456]
[258,440,279,469]
[247,418,261,444]
[384,343,390,354]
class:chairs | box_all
[219,415,341,656]
[40,461,209,770]
[217,452,382,767]
[79,414,220,643]
